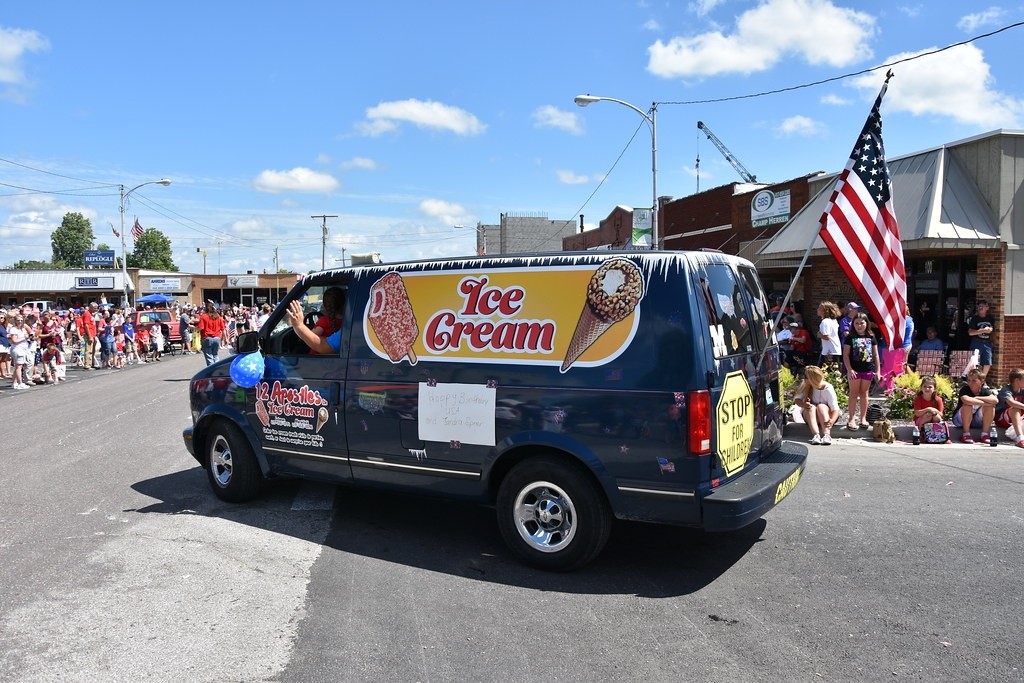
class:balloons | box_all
[230,346,264,387]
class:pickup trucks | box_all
[114,310,182,352]
[19,300,70,316]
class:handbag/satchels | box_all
[920,421,949,443]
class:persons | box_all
[42,343,62,385]
[793,365,842,443]
[83,302,98,373]
[42,312,65,384]
[180,306,197,356]
[32,326,56,379]
[968,299,994,385]
[770,295,915,395]
[10,315,34,390]
[843,312,881,432]
[0,312,13,379]
[0,300,290,351]
[199,297,223,367]
[912,367,1024,448]
[104,316,164,372]
[285,284,350,358]
[914,327,944,353]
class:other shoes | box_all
[0,372,66,389]
[859,423,869,427]
[72,350,195,371]
[847,421,858,430]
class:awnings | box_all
[751,150,1000,263]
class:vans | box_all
[181,246,809,572]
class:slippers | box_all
[980,435,991,444]
[961,435,973,443]
[1015,440,1024,448]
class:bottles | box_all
[912,426,920,445]
[990,427,998,447]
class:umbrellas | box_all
[137,291,175,309]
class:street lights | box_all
[573,94,660,249]
[453,224,487,255]
[120,177,173,306]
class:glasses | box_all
[804,375,808,379]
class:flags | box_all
[816,80,906,352]
[130,219,144,242]
[227,318,237,342]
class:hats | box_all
[847,302,861,310]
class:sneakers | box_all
[812,434,821,444]
[822,433,831,444]
